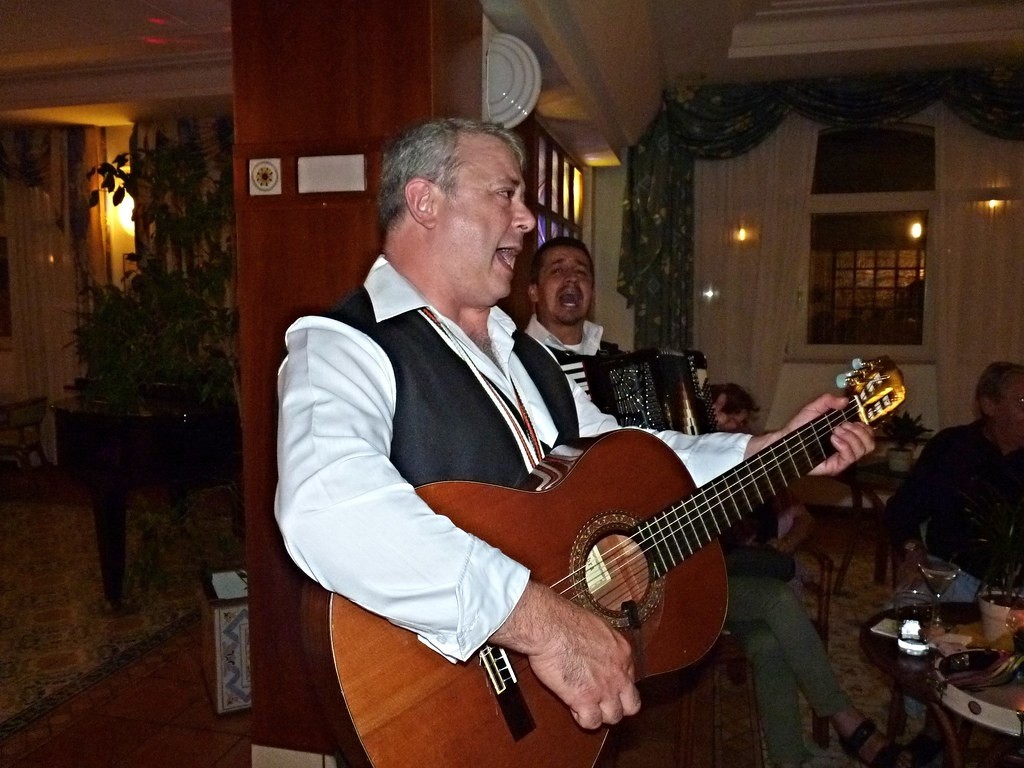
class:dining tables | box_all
[859,595,1024,766]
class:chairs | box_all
[0,392,57,505]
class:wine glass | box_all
[919,561,960,628]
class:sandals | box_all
[841,718,918,768]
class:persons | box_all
[718,577,891,767]
[706,382,762,436]
[719,487,824,598]
[717,536,797,585]
[273,114,878,733]
[881,358,1023,767]
[504,231,742,445]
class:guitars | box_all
[301,356,906,767]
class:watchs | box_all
[900,540,926,561]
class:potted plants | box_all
[880,410,935,472]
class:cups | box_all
[892,592,936,657]
